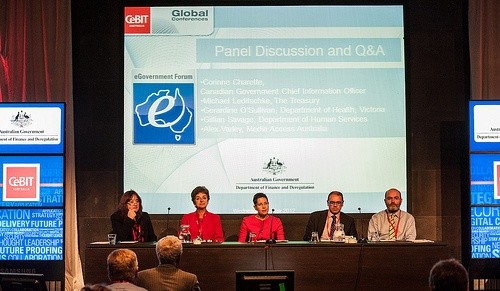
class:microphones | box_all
[167,207,170,235]
[265,208,276,244]
[358,208,367,243]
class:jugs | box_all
[333,224,345,242]
[179,225,192,243]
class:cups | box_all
[108,234,116,245]
[249,232,256,244]
[369,230,379,242]
[311,232,319,243]
[345,235,357,243]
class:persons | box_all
[304,191,359,240]
[238,193,285,243]
[428,260,469,291]
[107,249,147,291]
[368,189,417,241]
[179,186,224,242]
[82,284,111,291]
[110,190,156,241]
[139,235,197,291]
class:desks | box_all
[85,241,448,291]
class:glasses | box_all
[127,202,140,206]
[328,201,344,204]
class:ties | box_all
[389,215,396,240]
[330,216,337,240]
